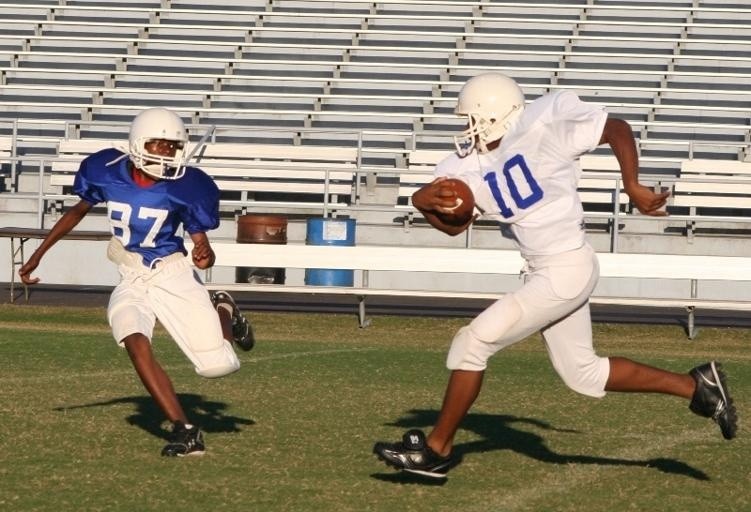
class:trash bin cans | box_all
[236,214,355,286]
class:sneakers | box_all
[373,429,450,479]
[162,420,205,457]
[210,291,254,352]
[689,360,737,440]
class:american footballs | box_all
[437,179,474,226]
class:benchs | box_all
[0,3,750,340]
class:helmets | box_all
[126,108,188,180]
[454,73,524,156]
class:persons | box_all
[372,71,741,486]
[16,107,255,459]
